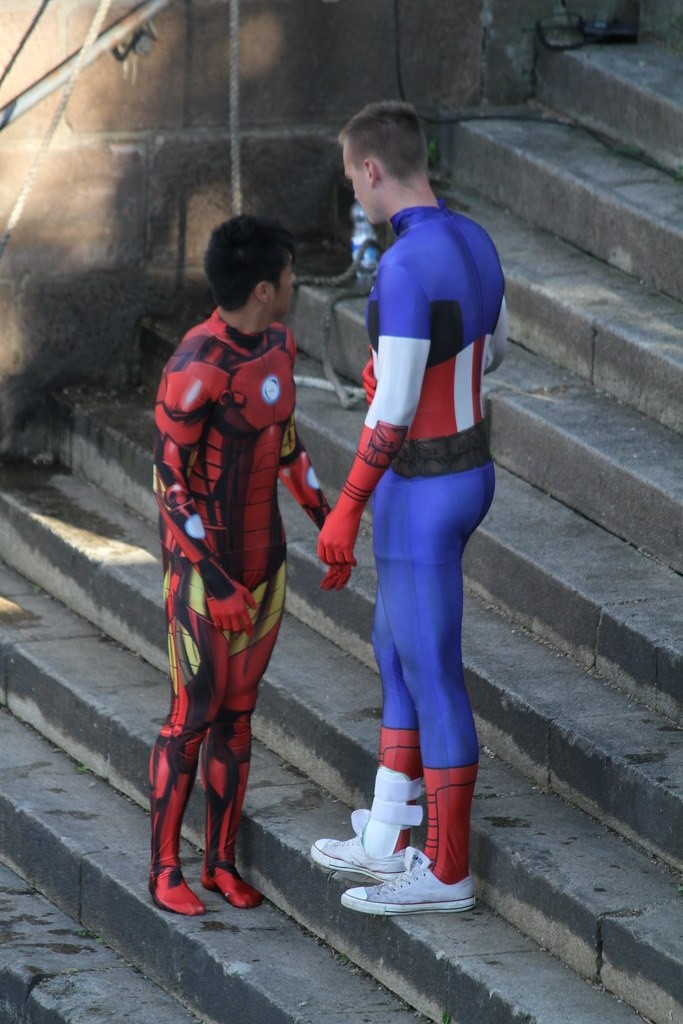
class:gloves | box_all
[360,345,378,405]
[320,564,351,591]
[317,506,356,567]
[206,578,257,636]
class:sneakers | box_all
[341,847,477,915]
[311,809,408,882]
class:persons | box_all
[317,102,510,915]
[148,216,353,916]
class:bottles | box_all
[350,204,380,291]
